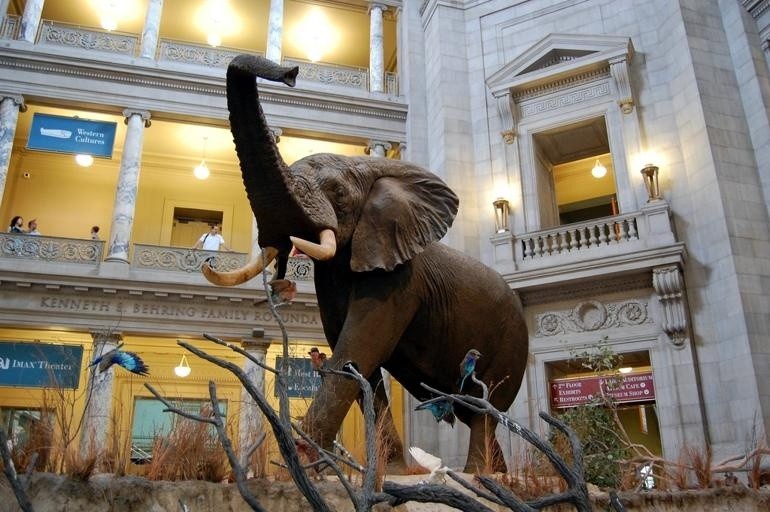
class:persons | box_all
[26,221,41,257]
[90,226,100,261]
[7,216,37,256]
[192,224,232,269]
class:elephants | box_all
[201,54,528,475]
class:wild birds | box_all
[414,399,455,428]
[86,348,151,378]
[308,348,326,379]
[456,349,484,393]
[253,280,297,308]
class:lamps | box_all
[492,191,511,233]
[640,155,663,203]
[174,355,191,377]
[592,160,606,178]
[195,158,210,180]
